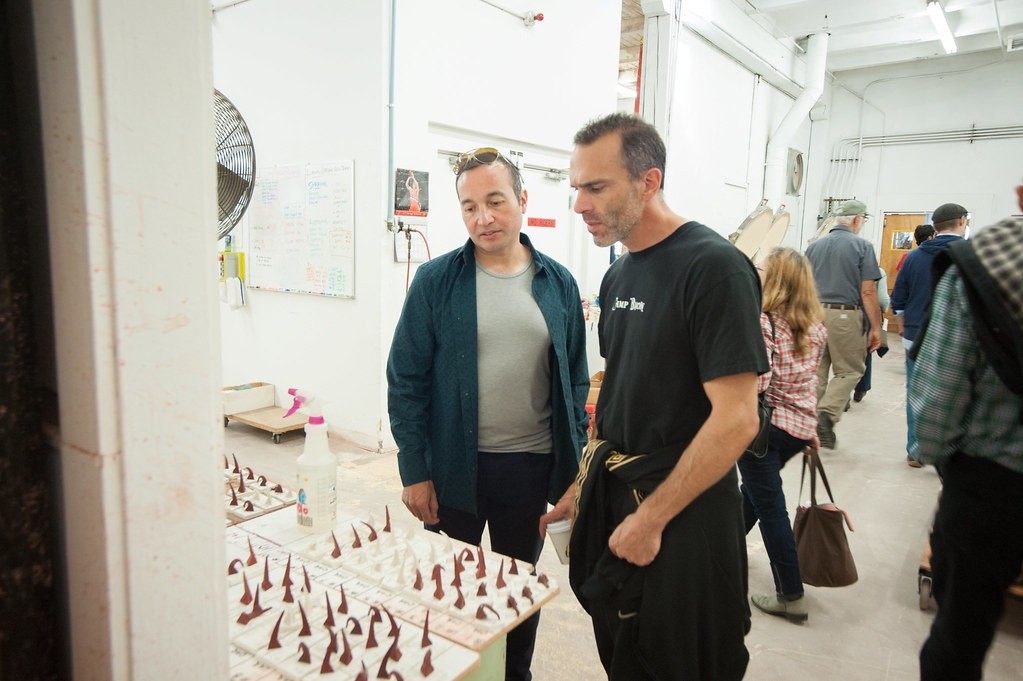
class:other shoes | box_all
[844,399,850,412]
[854,391,866,402]
[817,412,836,449]
[907,455,921,468]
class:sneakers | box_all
[751,594,808,621]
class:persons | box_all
[386,148,590,681]
[914,225,935,246]
[569,113,774,681]
[889,204,969,468]
[907,179,1023,681]
[804,199,883,448]
[736,246,831,623]
[844,265,890,410]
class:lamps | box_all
[927,0,957,54]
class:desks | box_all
[224,458,560,681]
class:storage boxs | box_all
[222,382,274,415]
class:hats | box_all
[932,203,969,224]
[836,199,867,215]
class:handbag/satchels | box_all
[792,446,858,587]
[877,326,889,358]
[746,312,775,459]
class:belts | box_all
[823,304,860,310]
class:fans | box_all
[214,85,256,244]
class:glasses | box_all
[453,147,524,184]
[862,216,869,222]
